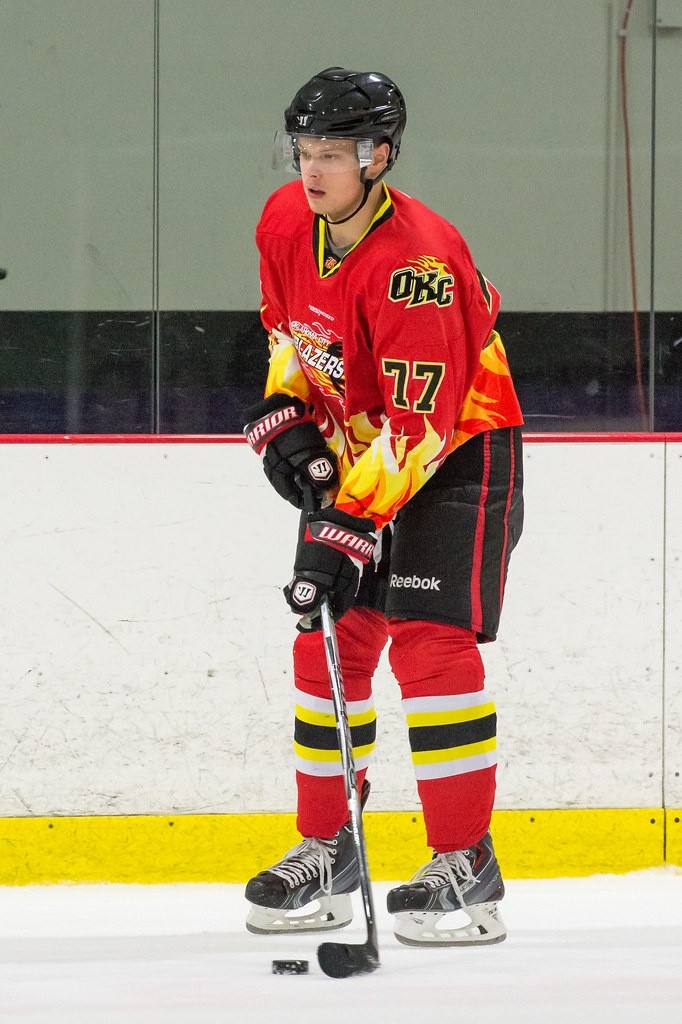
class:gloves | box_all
[283,508,379,632]
[243,391,336,513]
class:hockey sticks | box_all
[294,470,382,980]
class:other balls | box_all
[270,959,310,976]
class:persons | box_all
[240,66,525,946]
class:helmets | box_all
[284,66,406,166]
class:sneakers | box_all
[387,832,507,947]
[245,778,372,935]
